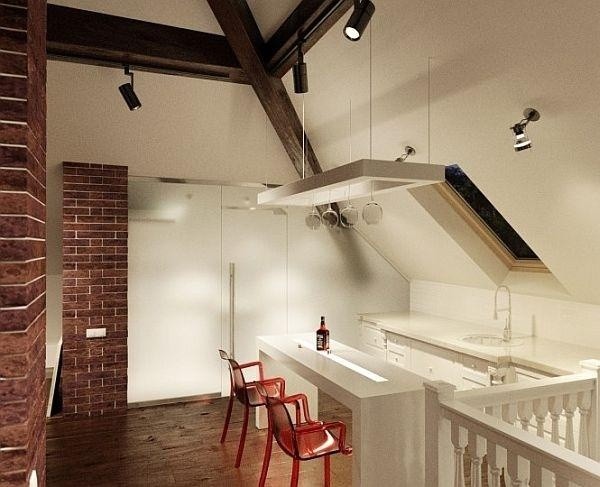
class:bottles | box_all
[315,315,331,353]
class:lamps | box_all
[291,21,309,95]
[508,106,542,152]
[341,0,376,42]
[117,63,142,112]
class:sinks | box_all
[459,333,522,350]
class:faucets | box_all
[493,285,517,341]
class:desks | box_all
[258,332,426,487]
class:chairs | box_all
[219,349,301,469]
[256,394,352,487]
